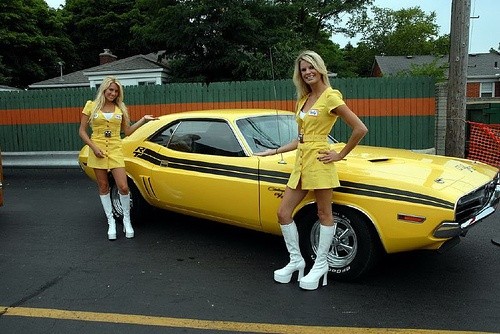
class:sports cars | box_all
[79,109,496,279]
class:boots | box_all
[298,223,337,291]
[100,193,117,239]
[118,191,134,238]
[274,221,305,284]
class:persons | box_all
[78,76,159,240]
[252,50,369,290]
